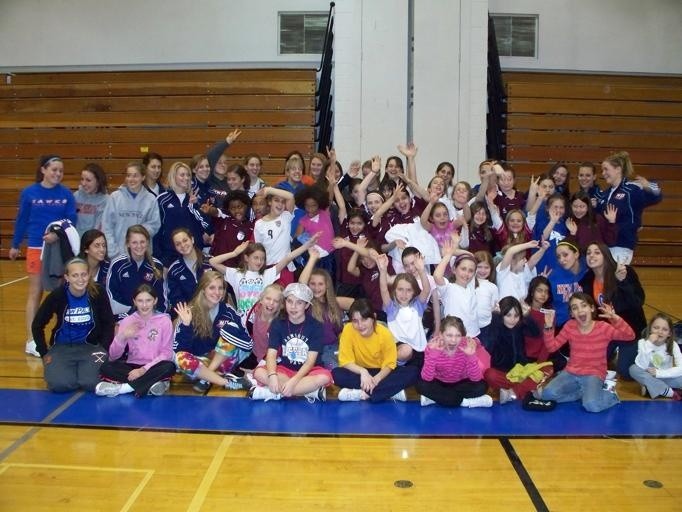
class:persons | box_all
[8,127,682,414]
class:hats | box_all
[283,282,313,303]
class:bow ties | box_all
[26,340,42,357]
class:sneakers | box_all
[603,380,616,391]
[606,370,616,379]
[469,394,493,408]
[150,380,171,395]
[95,382,122,396]
[193,380,211,392]
[305,386,327,403]
[223,373,253,390]
[248,386,283,402]
[500,388,512,404]
[640,385,648,396]
[391,388,407,403]
[338,387,363,401]
[420,394,435,406]
[672,388,681,400]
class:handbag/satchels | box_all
[522,391,556,411]
